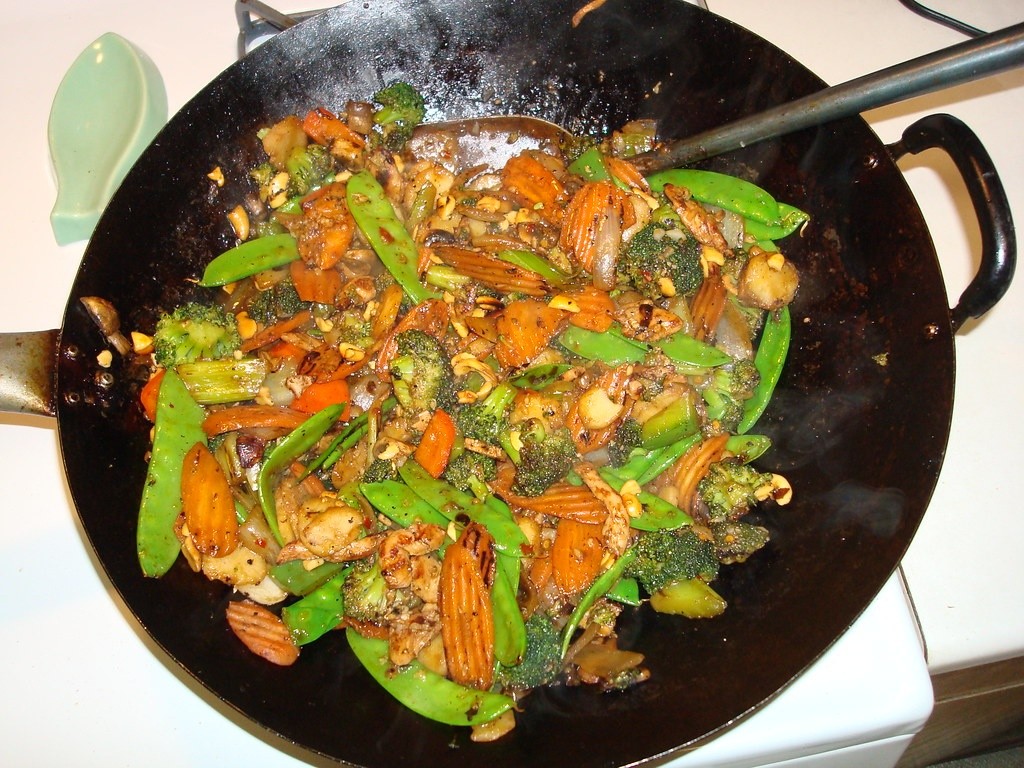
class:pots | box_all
[0,0,1017,768]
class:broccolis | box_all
[151,81,773,693]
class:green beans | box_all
[136,169,812,731]
